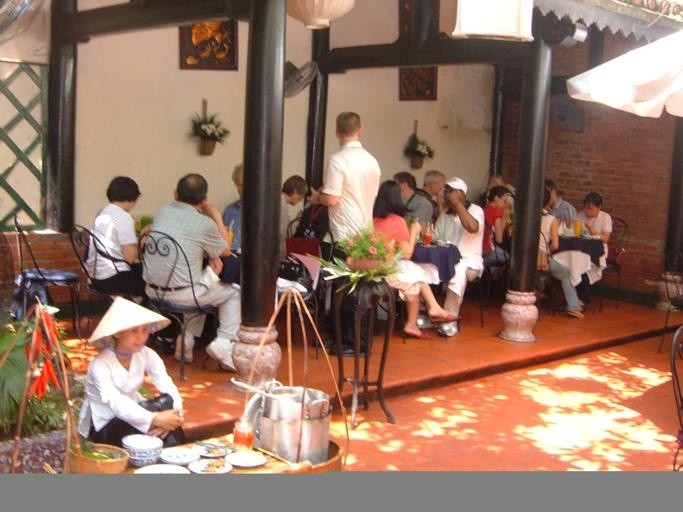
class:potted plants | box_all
[402,133,434,156]
[188,98,230,145]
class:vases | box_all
[349,252,379,271]
[411,156,423,168]
[199,137,216,155]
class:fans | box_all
[283,57,318,100]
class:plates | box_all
[136,440,269,474]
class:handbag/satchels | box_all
[277,261,313,288]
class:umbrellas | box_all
[564,23,683,125]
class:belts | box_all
[148,283,186,292]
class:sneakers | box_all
[438,323,458,336]
[417,315,434,329]
[205,339,236,372]
[174,335,194,363]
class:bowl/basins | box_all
[66,442,129,473]
[122,434,164,467]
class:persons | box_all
[142,172,245,373]
[221,165,245,283]
[373,171,516,337]
[535,179,613,318]
[75,291,188,446]
[278,175,328,314]
[81,174,148,300]
[307,109,383,361]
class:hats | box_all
[442,177,467,196]
[90,297,172,349]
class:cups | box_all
[226,229,233,250]
[573,218,583,236]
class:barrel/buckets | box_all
[260,379,332,464]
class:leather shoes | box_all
[329,344,370,356]
[312,336,343,348]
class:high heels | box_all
[427,312,462,324]
[401,330,431,341]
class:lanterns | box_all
[451,2,536,44]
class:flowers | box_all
[334,224,398,260]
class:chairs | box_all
[286,216,334,361]
[476,215,629,330]
[7,210,228,384]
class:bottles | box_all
[233,415,253,451]
[423,221,433,248]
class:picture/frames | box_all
[400,67,438,101]
[177,22,240,71]
